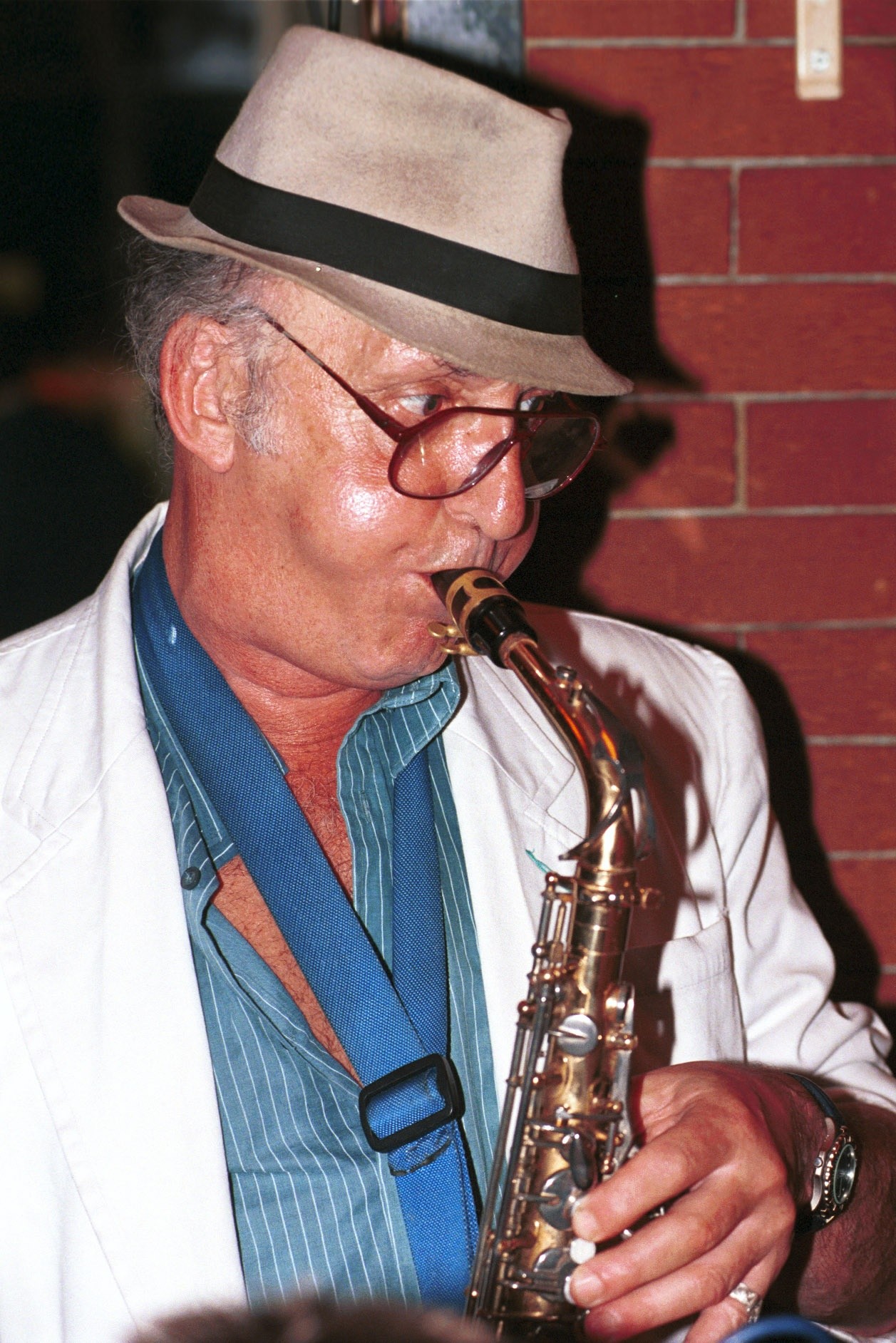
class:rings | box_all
[729,1282,762,1323]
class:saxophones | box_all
[427,565,654,1343]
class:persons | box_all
[0,25,896,1343]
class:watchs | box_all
[785,1072,856,1238]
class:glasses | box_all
[219,305,609,502]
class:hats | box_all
[117,26,635,396]
